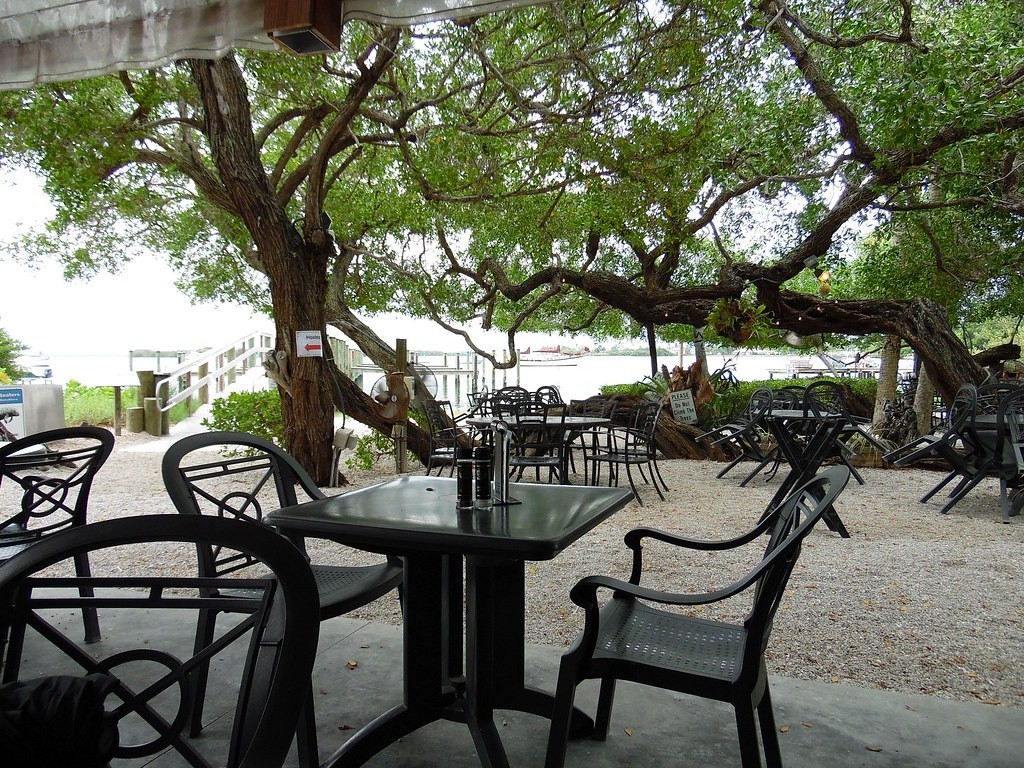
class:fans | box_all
[369,361,437,475]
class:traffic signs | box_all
[296,330,323,357]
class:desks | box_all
[754,400,812,409]
[963,415,1024,517]
[263,472,635,768]
[466,416,611,487]
[748,409,842,482]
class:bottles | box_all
[456,447,473,510]
[475,448,493,511]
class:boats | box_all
[518,343,591,367]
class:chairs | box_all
[0,425,102,685]
[162,431,403,768]
[693,380,890,486]
[0,514,321,768]
[882,382,1024,524]
[420,386,670,508]
[544,465,851,768]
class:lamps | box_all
[803,254,830,282]
